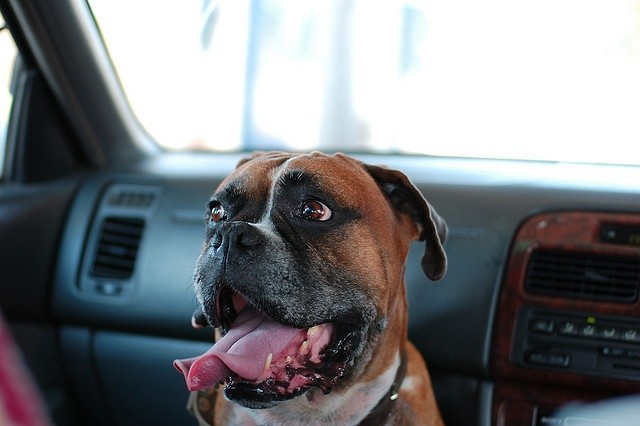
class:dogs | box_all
[173,150,448,426]
